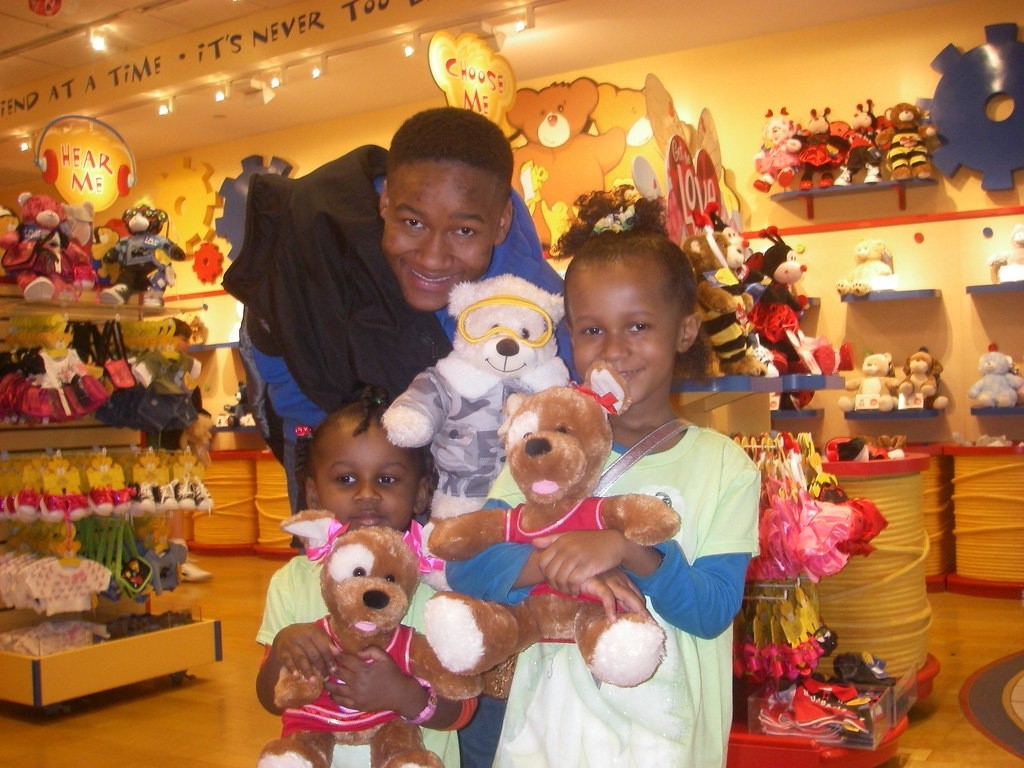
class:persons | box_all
[161,316,212,582]
[224,107,580,768]
[258,386,461,768]
[445,182,761,768]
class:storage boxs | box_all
[748,663,918,751]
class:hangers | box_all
[5,313,73,358]
[119,319,179,360]
[727,430,838,504]
[0,519,81,568]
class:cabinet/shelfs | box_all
[767,179,1023,419]
[185,342,257,432]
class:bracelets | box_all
[400,677,436,724]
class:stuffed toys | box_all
[754,99,940,193]
[683,210,854,419]
[0,191,187,310]
[225,381,250,426]
[953,432,1024,446]
[838,346,949,412]
[258,509,486,768]
[421,360,680,687]
[382,273,574,593]
[969,343,1023,407]
[179,413,212,469]
[836,238,893,297]
[826,435,906,461]
[988,224,1024,283]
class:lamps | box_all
[404,32,421,58]
[312,55,329,79]
[271,67,286,88]
[158,95,175,115]
[216,81,231,102]
[515,7,535,34]
[85,26,109,54]
